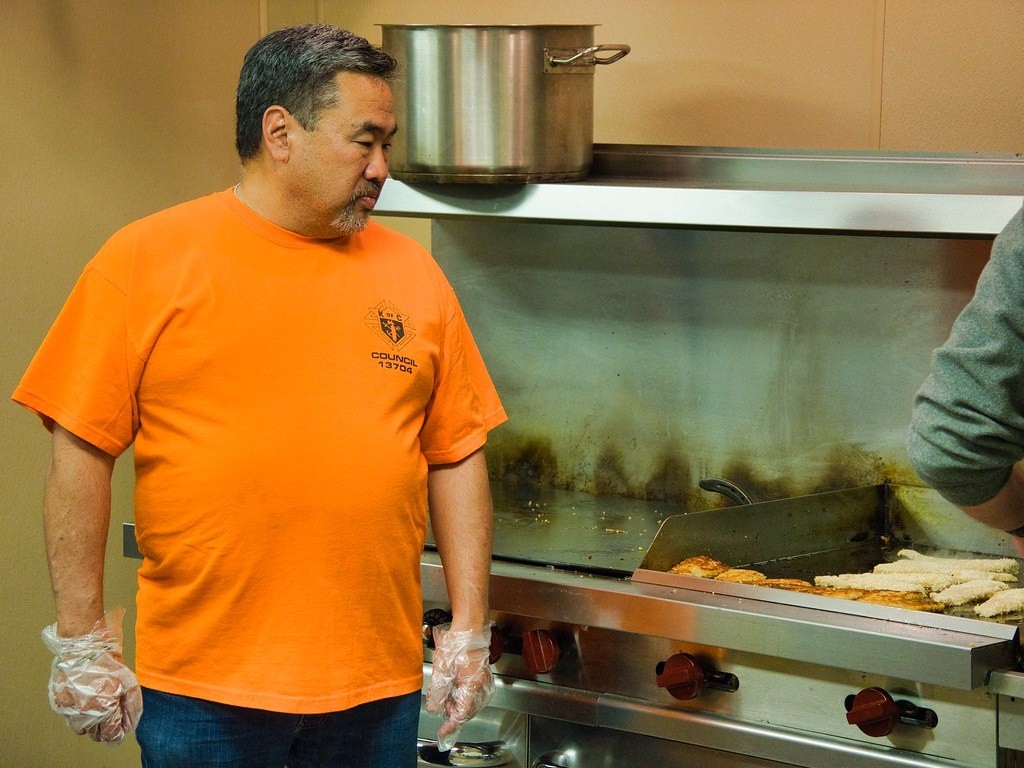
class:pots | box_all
[372,24,631,186]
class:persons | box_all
[905,210,1024,564]
[11,23,509,768]
